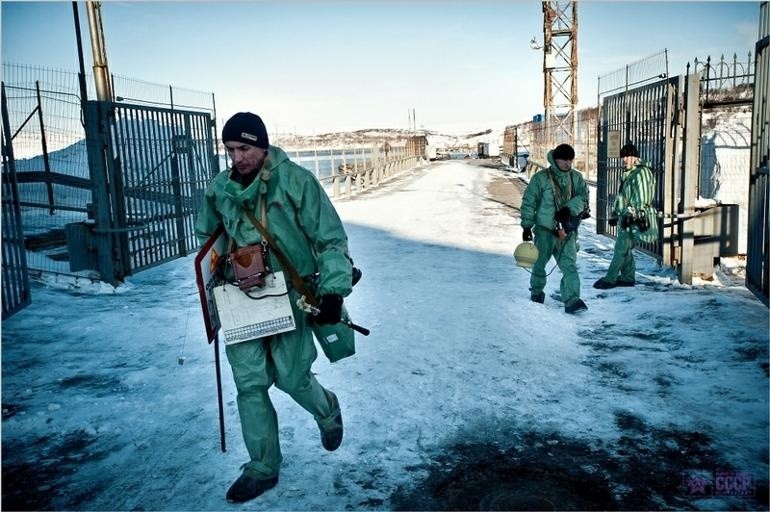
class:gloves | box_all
[553,206,572,223]
[316,291,346,327]
[607,216,620,227]
[521,226,533,241]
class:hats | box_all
[219,111,272,150]
[620,143,640,158]
[553,143,575,160]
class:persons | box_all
[193,111,358,503]
[520,142,588,315]
[592,143,655,289]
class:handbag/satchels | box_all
[212,270,298,347]
[630,206,660,243]
[311,301,357,364]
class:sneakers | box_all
[531,292,545,303]
[593,277,635,289]
[565,299,588,314]
[321,414,346,453]
[224,475,279,506]
